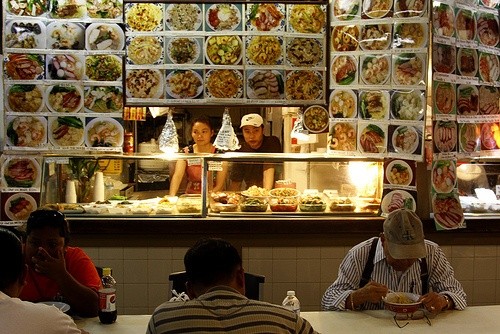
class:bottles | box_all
[282,291,300,316]
[98,268,117,325]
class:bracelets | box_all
[350,292,361,311]
[56,278,73,295]
[442,293,450,311]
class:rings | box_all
[430,305,435,311]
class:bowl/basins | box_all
[40,302,70,315]
[84,196,202,214]
[211,188,380,212]
[205,35,243,65]
[461,202,500,213]
[382,292,424,313]
[141,144,158,153]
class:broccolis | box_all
[89,87,119,110]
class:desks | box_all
[75,304,500,334]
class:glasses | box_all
[394,310,431,328]
[29,209,66,220]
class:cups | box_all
[65,181,77,204]
[291,144,301,153]
[93,172,104,203]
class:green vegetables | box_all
[88,56,118,77]
[391,164,407,171]
[48,64,66,80]
[363,56,374,68]
[435,43,497,97]
[248,3,259,20]
[276,74,285,94]
[94,31,112,44]
[50,85,76,94]
[393,25,403,48]
[436,192,453,198]
[395,53,416,65]
[360,99,372,118]
[368,124,385,139]
[346,5,359,19]
[8,0,59,15]
[434,3,494,20]
[9,83,36,94]
[8,197,23,212]
[436,160,451,167]
[96,10,110,17]
[6,122,18,145]
[4,175,34,187]
[58,116,83,128]
[338,70,356,85]
[92,137,112,147]
[27,54,45,65]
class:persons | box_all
[146,236,322,334]
[20,209,103,318]
[167,118,229,196]
[320,206,469,313]
[0,227,90,334]
[229,113,283,191]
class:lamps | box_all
[213,106,241,151]
[290,107,310,140]
[159,108,178,151]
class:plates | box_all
[125,2,325,100]
[6,115,124,148]
[382,159,416,215]
[433,46,500,152]
[303,90,426,154]
[1,157,42,220]
[432,160,465,229]
[2,53,123,81]
[5,84,123,113]
[433,0,499,47]
[4,0,124,51]
[139,160,169,172]
[331,0,428,85]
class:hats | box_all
[239,113,264,128]
[384,209,427,260]
